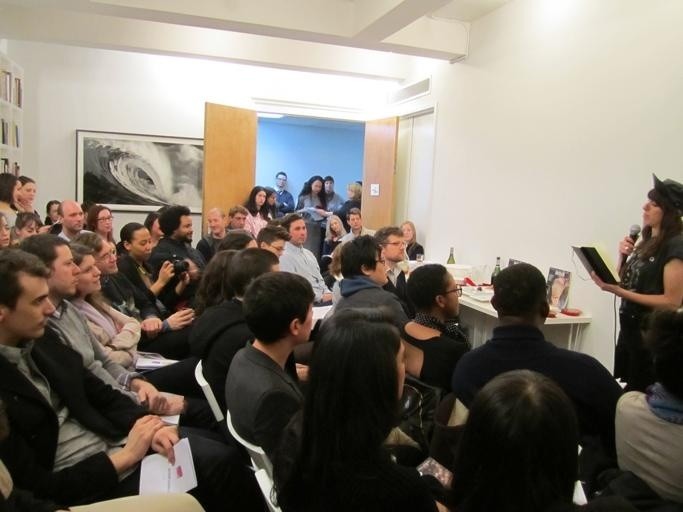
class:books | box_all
[1,70,22,178]
[571,242,621,286]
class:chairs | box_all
[302,219,323,260]
[250,467,285,512]
[403,371,445,441]
[194,356,236,448]
[223,406,276,480]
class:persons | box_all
[1,173,40,225]
[451,263,622,467]
[273,172,362,233]
[0,186,471,512]
[589,178,683,399]
[448,368,578,512]
[614,307,683,512]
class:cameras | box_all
[167,259,189,282]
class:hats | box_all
[650,172,683,217]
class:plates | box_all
[545,312,556,317]
[561,308,580,315]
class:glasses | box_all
[96,216,115,222]
[380,239,408,249]
[442,283,463,298]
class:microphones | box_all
[620,224,641,273]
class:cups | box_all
[416,254,424,262]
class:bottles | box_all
[490,256,501,284]
[447,246,455,264]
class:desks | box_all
[453,281,592,357]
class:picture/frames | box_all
[74,126,203,216]
